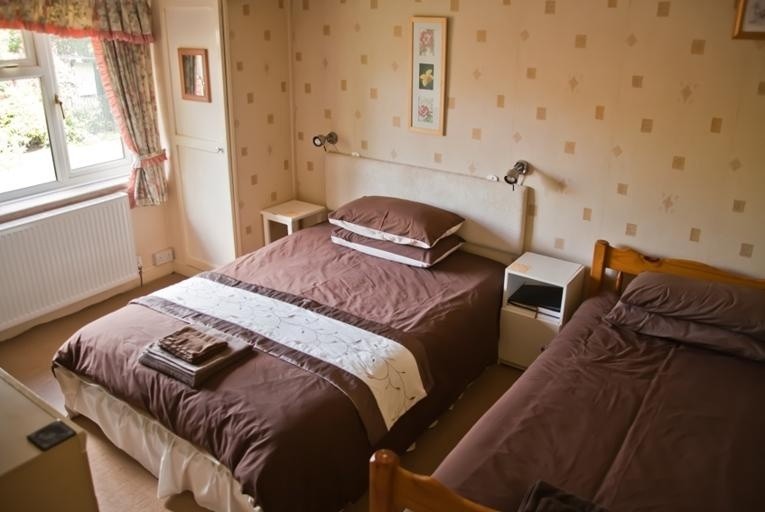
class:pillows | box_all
[330,227,466,269]
[328,195,465,250]
[605,300,765,364]
[620,271,765,339]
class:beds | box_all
[50,151,527,512]
[367,239,765,512]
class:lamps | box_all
[504,160,528,185]
[313,131,337,147]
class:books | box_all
[508,284,537,313]
[537,286,563,318]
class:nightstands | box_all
[499,251,585,371]
[260,199,326,246]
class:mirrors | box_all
[177,48,211,103]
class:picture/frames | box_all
[732,0,765,40]
[406,15,448,137]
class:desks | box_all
[0,368,100,512]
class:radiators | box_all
[0,192,140,333]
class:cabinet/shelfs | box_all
[157,0,295,272]
